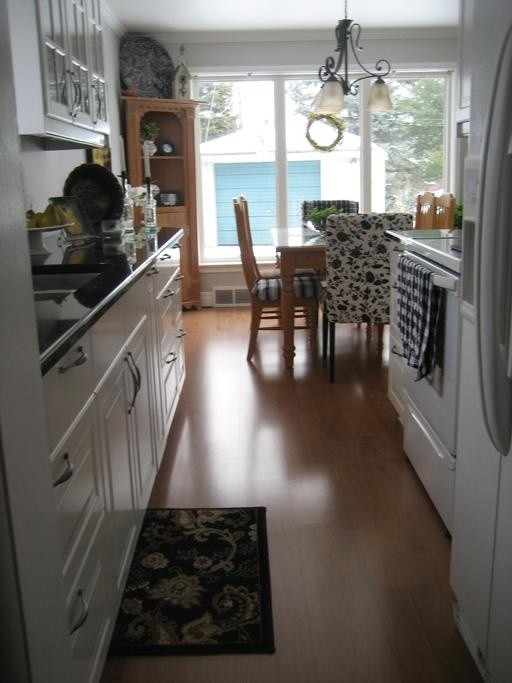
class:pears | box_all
[28,204,66,228]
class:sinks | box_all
[30,263,111,294]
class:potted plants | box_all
[141,118,161,156]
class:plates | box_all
[24,220,76,231]
[63,162,124,231]
[47,194,97,242]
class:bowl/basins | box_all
[156,190,183,207]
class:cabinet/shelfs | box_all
[8,0,93,147]
[85,1,111,136]
[44,334,120,683]
[119,93,206,310]
[145,239,187,439]
[91,275,159,593]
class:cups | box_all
[121,230,159,264]
[120,197,157,228]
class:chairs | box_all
[415,191,455,230]
[234,195,412,384]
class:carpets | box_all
[108,505,277,658]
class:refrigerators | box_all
[449,1,512,682]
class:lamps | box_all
[305,0,396,116]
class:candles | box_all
[143,143,150,179]
[119,135,126,171]
